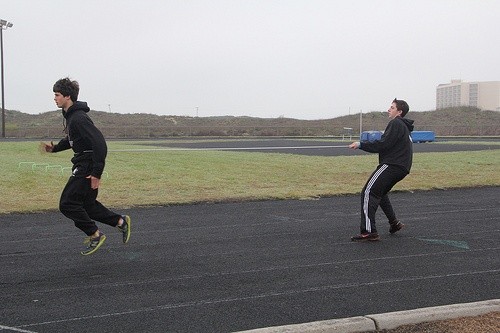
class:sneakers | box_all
[117,215,131,244]
[351,232,380,242]
[389,221,405,235]
[81,231,107,256]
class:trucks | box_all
[359,130,437,144]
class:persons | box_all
[348,98,414,242]
[38,76,131,255]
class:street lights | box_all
[0,18,13,137]
